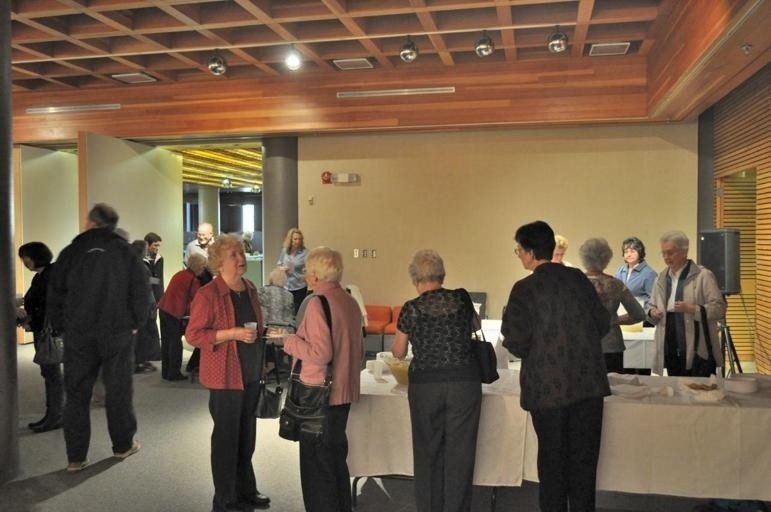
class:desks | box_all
[471,319,656,370]
[345,351,771,512]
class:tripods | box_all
[716,319,742,377]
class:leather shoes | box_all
[214,489,271,512]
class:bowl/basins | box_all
[384,358,410,393]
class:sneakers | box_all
[168,371,189,381]
[112,439,143,459]
[67,457,91,471]
[135,362,157,374]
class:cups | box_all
[618,297,645,332]
[243,322,259,344]
[15,293,24,310]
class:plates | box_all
[367,351,393,373]
[262,333,298,340]
[724,375,760,394]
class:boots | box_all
[27,373,65,434]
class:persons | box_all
[186,222,216,286]
[116,228,164,374]
[550,235,572,267]
[275,228,311,318]
[391,249,482,512]
[281,246,366,509]
[614,238,658,305]
[578,238,646,373]
[185,233,271,512]
[499,220,611,511]
[45,203,153,472]
[643,230,727,377]
[159,253,208,382]
[19,242,66,432]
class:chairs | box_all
[362,305,402,356]
[256,285,297,385]
[468,291,488,320]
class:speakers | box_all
[698,229,740,295]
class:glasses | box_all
[514,247,529,256]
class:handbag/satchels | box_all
[690,305,718,377]
[253,340,284,419]
[455,288,500,385]
[279,378,331,444]
